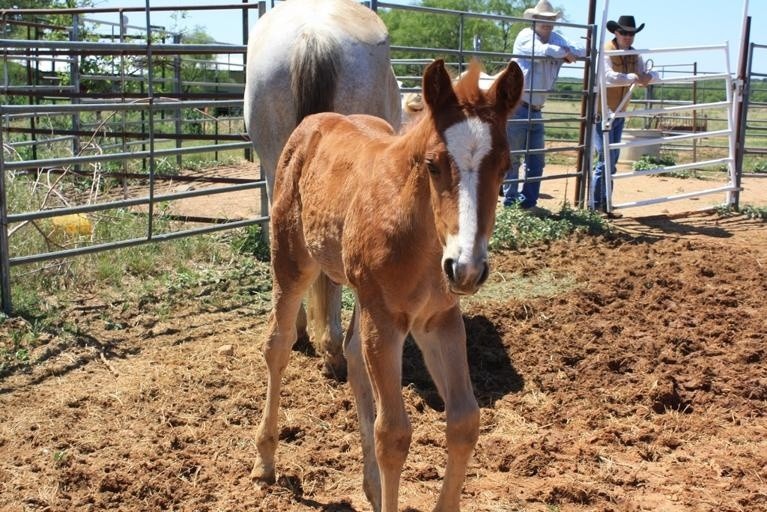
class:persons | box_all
[591,15,659,210]
[503,0,580,215]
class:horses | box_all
[242,0,403,382]
[250,55,523,511]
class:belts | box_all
[521,101,542,112]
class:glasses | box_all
[617,30,635,36]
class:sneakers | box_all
[528,206,552,217]
[595,208,622,219]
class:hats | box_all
[606,16,645,34]
[524,0,563,20]
